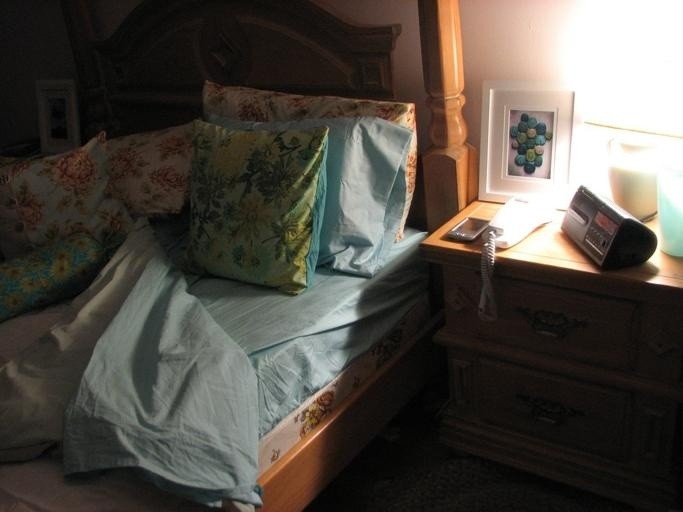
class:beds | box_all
[0,1,422,512]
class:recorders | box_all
[560,184,657,269]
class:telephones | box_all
[480,193,556,249]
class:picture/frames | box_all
[478,77,582,210]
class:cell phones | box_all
[448,216,492,241]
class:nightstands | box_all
[422,188,683,512]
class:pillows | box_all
[1,130,132,247]
[107,120,191,218]
[205,110,412,279]
[1,214,125,323]
[202,79,419,245]
[189,119,331,296]
